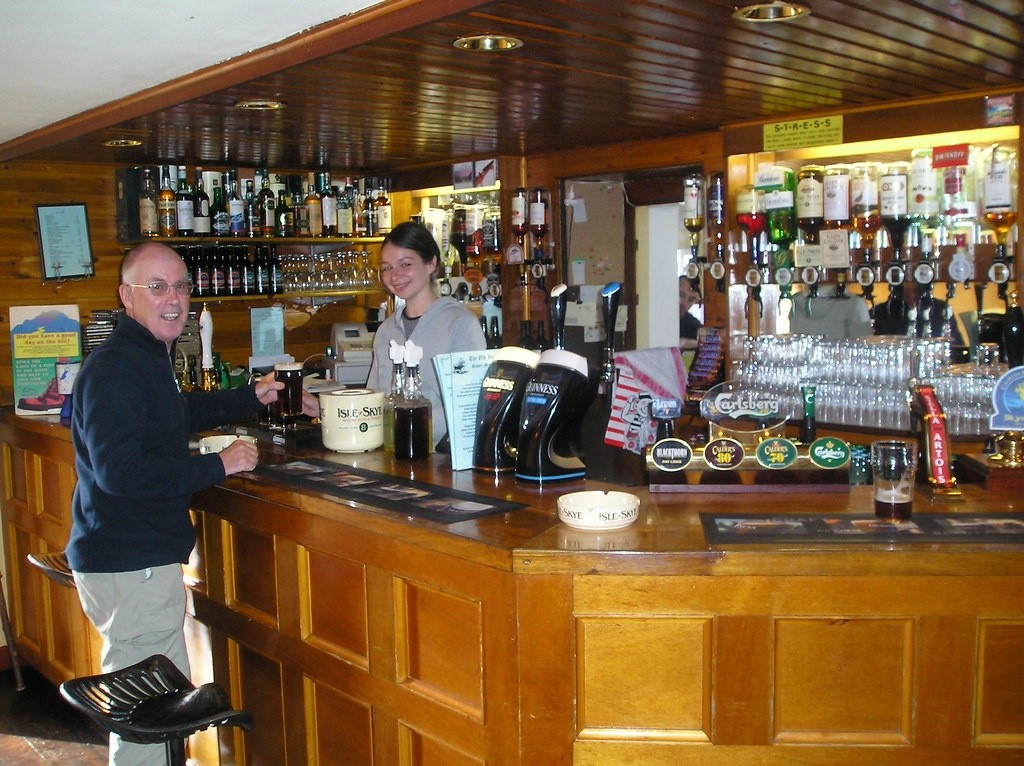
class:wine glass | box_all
[280,250,378,292]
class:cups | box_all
[729,332,1007,435]
[274,363,303,417]
[869,440,918,518]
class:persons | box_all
[868,281,957,339]
[62,243,286,766]
[301,221,487,454]
[679,275,704,352]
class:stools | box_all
[58,654,254,766]
[28,549,77,589]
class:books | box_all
[431,347,505,472]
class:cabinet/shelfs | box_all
[122,232,388,303]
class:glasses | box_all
[124,278,200,296]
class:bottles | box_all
[682,142,1019,298]
[137,165,393,236]
[173,245,285,297]
[384,364,431,462]
[445,185,555,357]
